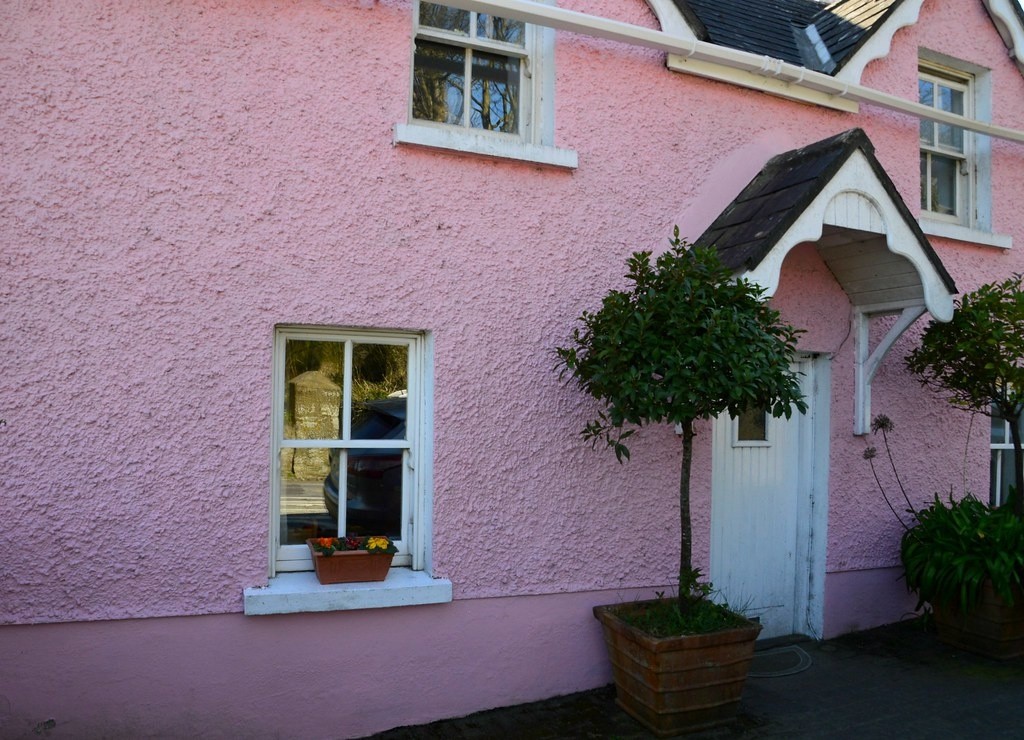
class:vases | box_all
[307,538,394,585]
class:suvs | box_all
[324,390,408,530]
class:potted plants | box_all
[863,414,1024,661]
[548,223,810,737]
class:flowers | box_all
[312,536,398,558]
